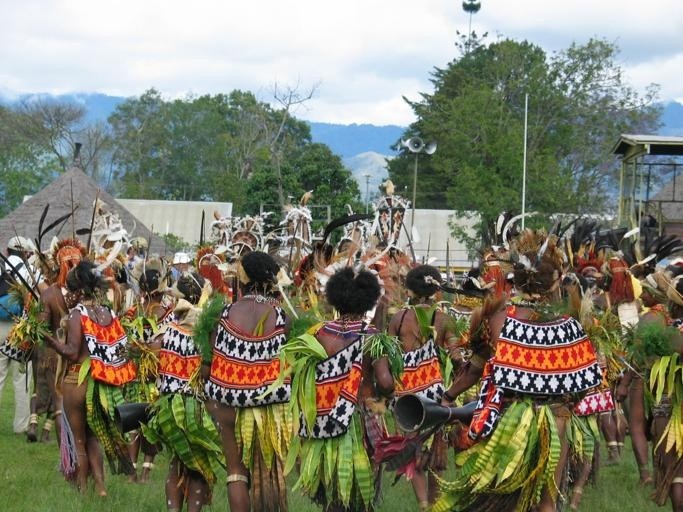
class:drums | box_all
[114,402,157,434]
[393,393,477,433]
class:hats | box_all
[172,252,191,264]
[127,237,148,254]
[4,255,24,271]
[7,236,28,252]
